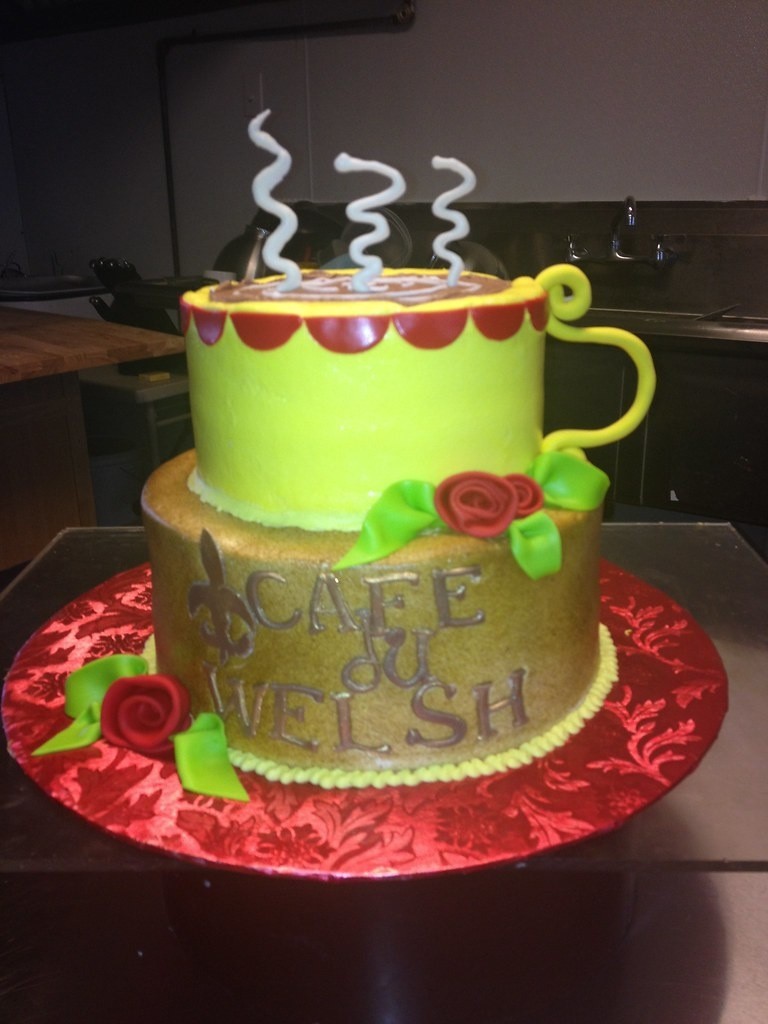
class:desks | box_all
[0,304,185,573]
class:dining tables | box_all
[0,524,768,1024]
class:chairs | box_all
[81,275,219,482]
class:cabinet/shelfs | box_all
[540,317,767,530]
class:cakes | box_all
[138,109,655,790]
